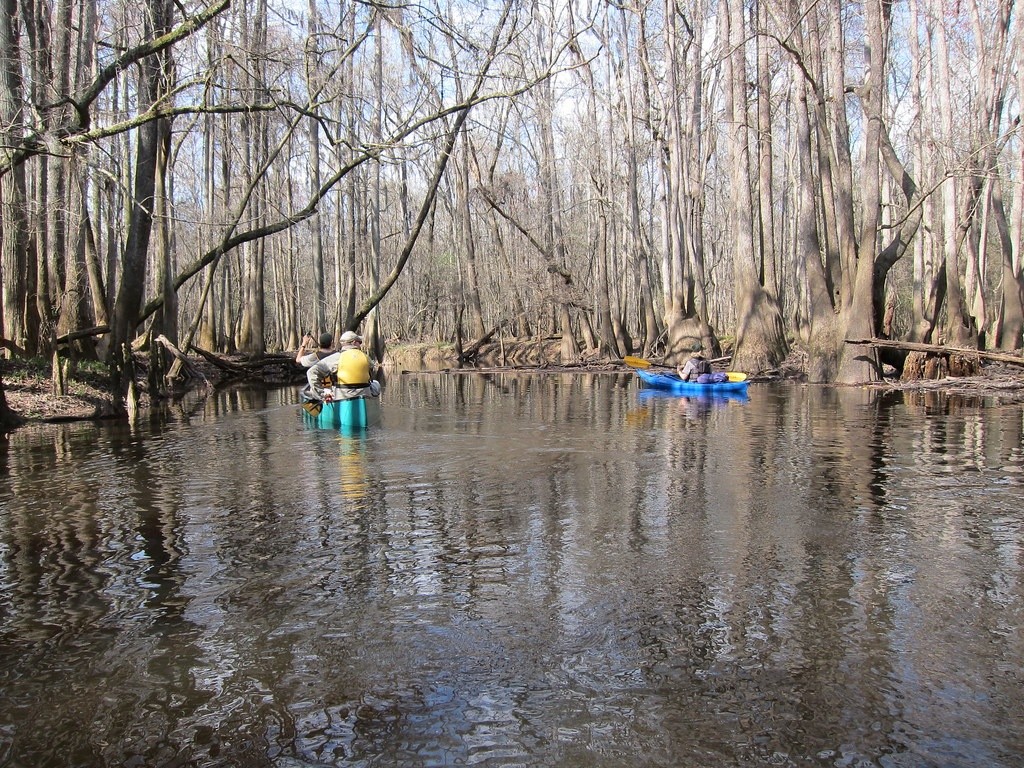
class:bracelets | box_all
[302,346,305,348]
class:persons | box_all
[677,344,712,382]
[296,334,381,401]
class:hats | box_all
[320,332,332,345]
[339,331,363,345]
[692,344,706,352]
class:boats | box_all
[301,383,380,428]
[637,368,752,393]
